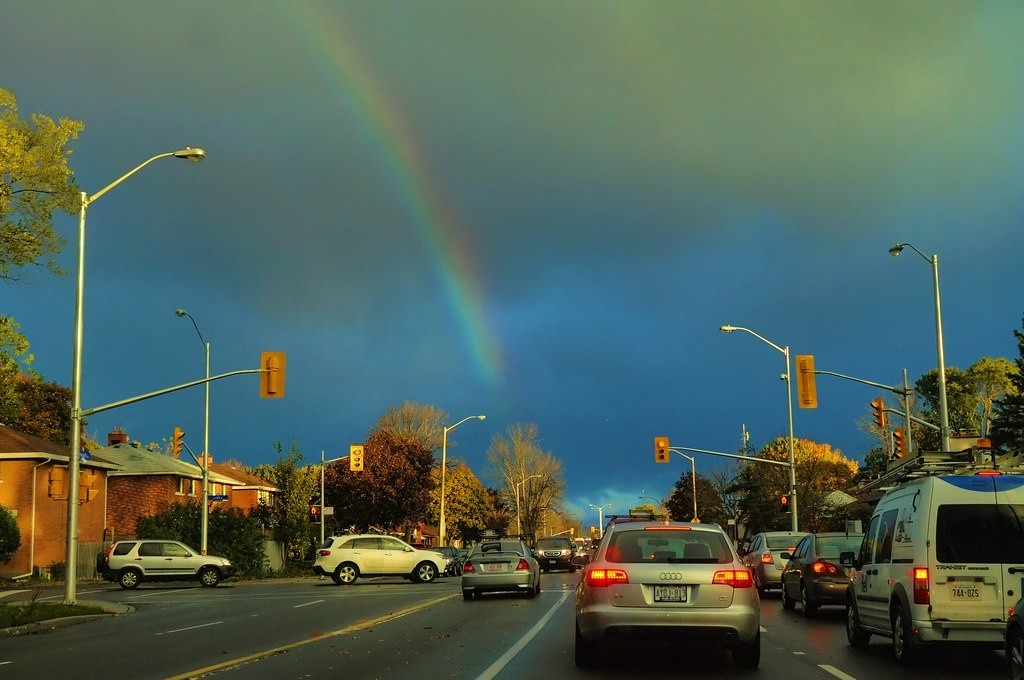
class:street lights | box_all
[440,415,486,547]
[516,474,542,541]
[889,243,951,452]
[175,309,210,555]
[719,325,798,532]
[62,148,206,605]
[588,503,612,539]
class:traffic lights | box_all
[349,445,364,470]
[174,427,185,459]
[654,436,670,463]
[870,397,885,430]
[892,427,907,459]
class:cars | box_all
[407,541,597,600]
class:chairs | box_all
[686,543,710,558]
[623,545,642,560]
[823,545,838,555]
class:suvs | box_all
[747,532,812,598]
[531,536,576,573]
[97,540,234,589]
[311,534,447,585]
[571,519,761,668]
[780,532,865,617]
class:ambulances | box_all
[839,439,1024,666]
